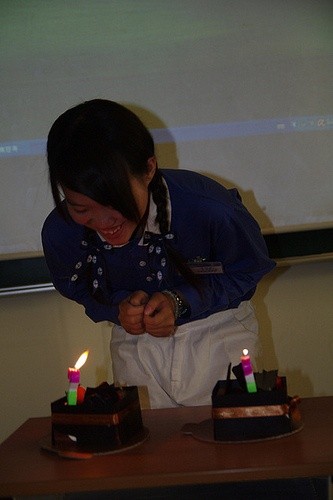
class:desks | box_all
[0,396,333,500]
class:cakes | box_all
[50,381,145,451]
[210,362,300,442]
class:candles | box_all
[67,350,88,405]
[240,349,257,393]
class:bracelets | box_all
[161,288,192,318]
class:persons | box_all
[41,99,277,410]
